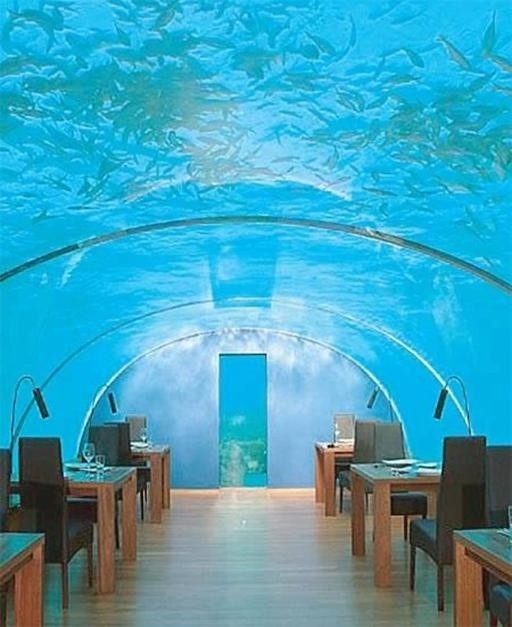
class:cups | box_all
[95,455,106,469]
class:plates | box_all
[381,458,419,466]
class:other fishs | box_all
[0,0,512,241]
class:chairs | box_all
[1,416,148,626]
[334,414,512,627]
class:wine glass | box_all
[83,442,96,464]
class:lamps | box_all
[9,376,117,453]
[365,376,470,434]
